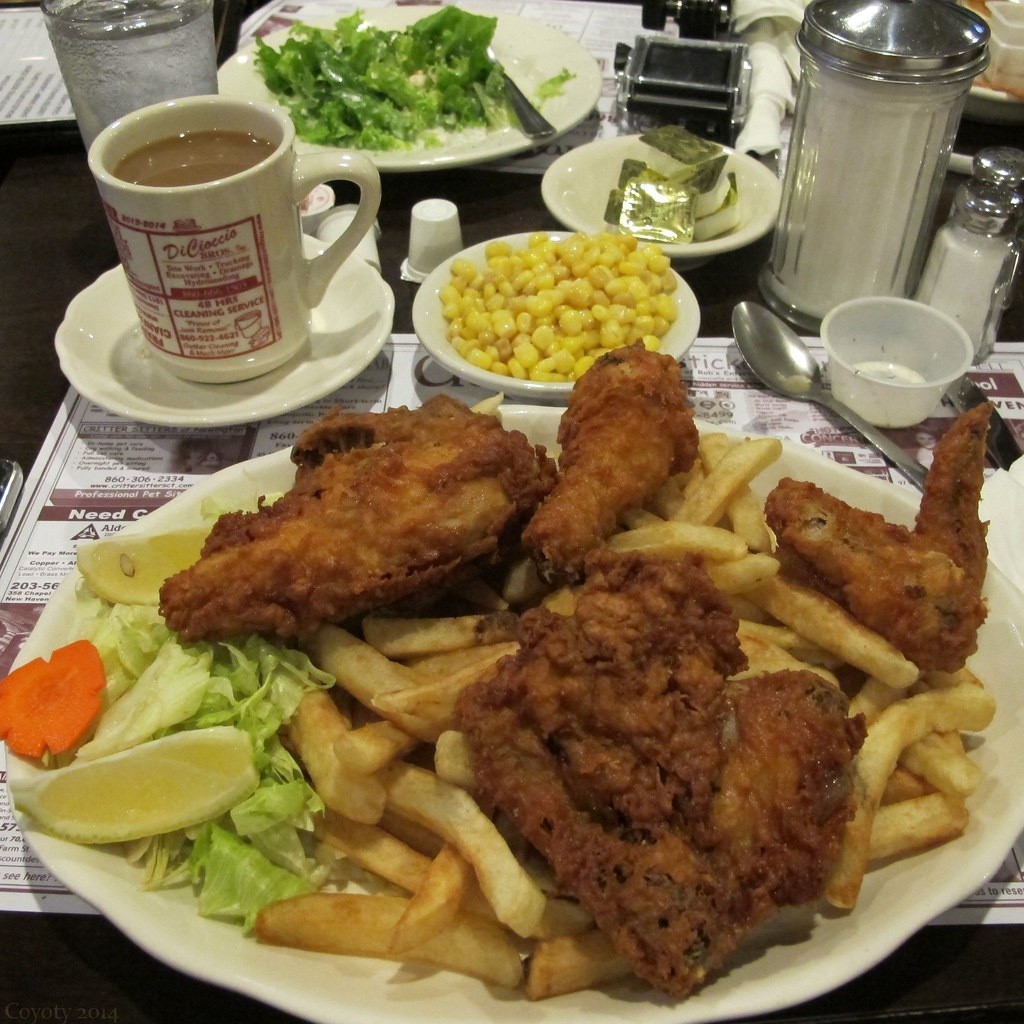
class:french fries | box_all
[255,389,998,1003]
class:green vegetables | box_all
[251,5,575,150]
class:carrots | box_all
[1,639,107,761]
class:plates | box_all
[6,398,1021,1024]
[52,232,398,428]
[218,5,602,173]
[410,229,700,400]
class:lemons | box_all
[76,529,205,606]
[11,725,260,845]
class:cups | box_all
[83,91,381,385]
[41,0,221,151]
[754,0,991,335]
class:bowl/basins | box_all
[542,131,785,269]
[818,298,974,431]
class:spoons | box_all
[730,296,930,492]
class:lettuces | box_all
[94,601,336,939]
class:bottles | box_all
[913,144,1022,362]
